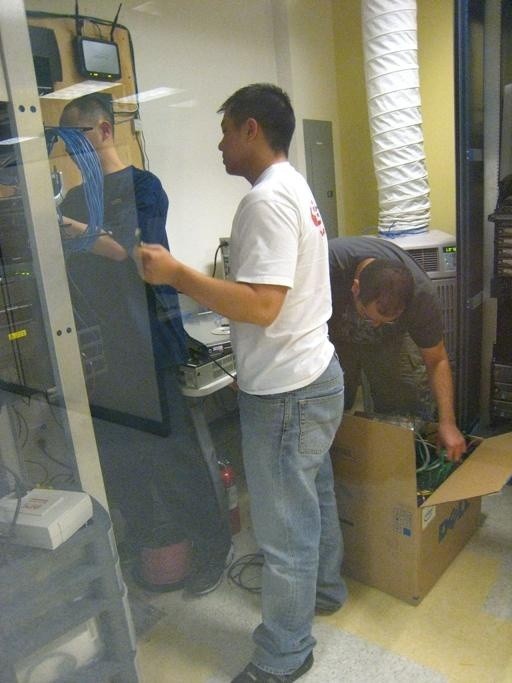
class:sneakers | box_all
[187,518,235,594]
[230,647,313,683]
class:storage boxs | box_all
[332,406,510,607]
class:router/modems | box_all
[72,0,121,81]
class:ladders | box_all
[0,0,140,683]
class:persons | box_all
[321,235,470,461]
[53,92,235,595]
[130,83,346,682]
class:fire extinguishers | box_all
[217,455,240,535]
